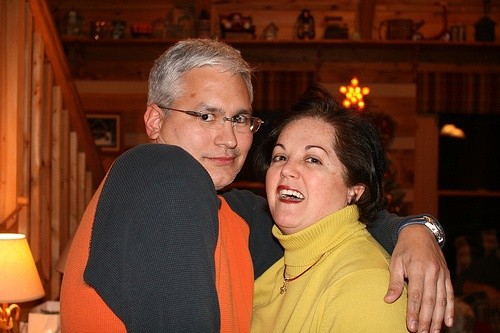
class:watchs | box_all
[397,215,444,246]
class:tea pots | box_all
[379,11,425,40]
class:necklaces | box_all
[280,253,324,294]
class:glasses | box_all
[158,106,264,133]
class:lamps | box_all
[0,232,46,333]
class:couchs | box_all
[454,230,500,326]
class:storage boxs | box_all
[27,300,60,333]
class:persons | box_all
[250,80,410,333]
[59,38,455,333]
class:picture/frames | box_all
[84,109,125,157]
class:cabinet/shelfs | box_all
[413,112,500,233]
